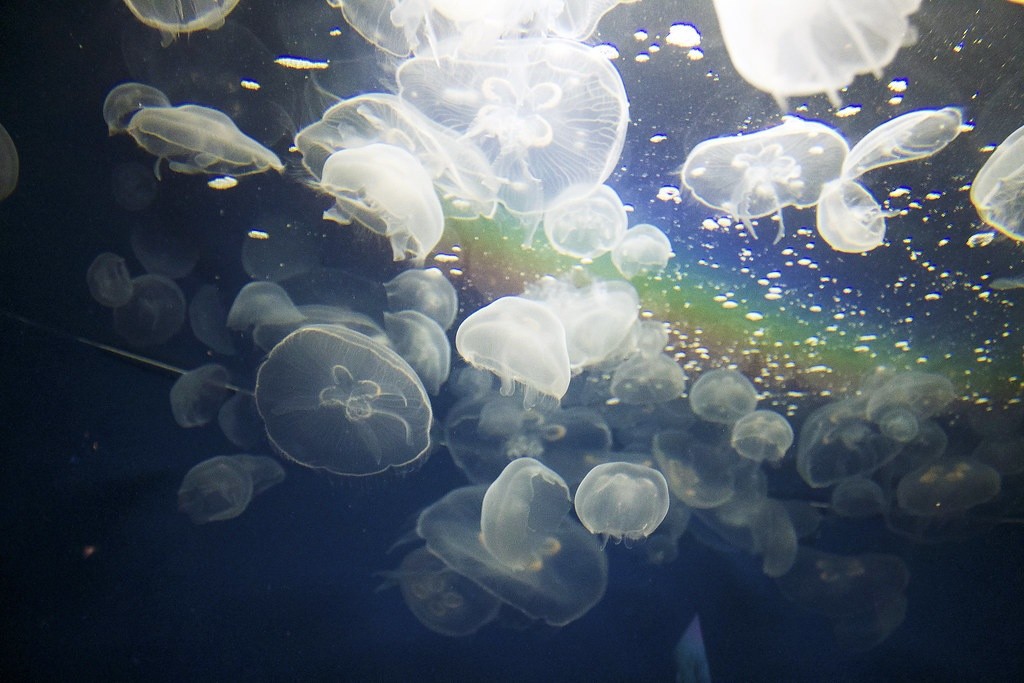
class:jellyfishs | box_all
[0,0,1024,639]
